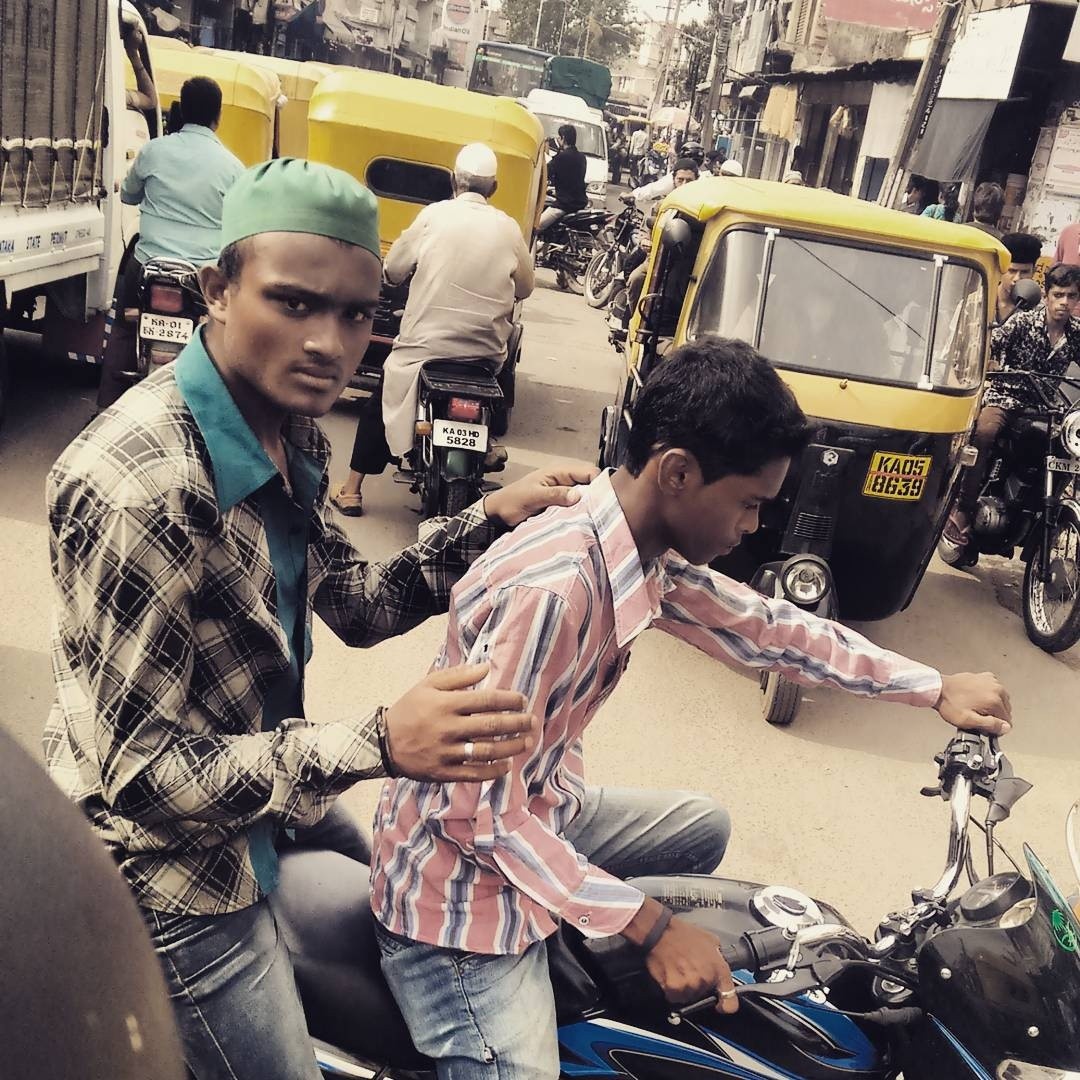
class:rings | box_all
[718,987,736,998]
[464,741,475,762]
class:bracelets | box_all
[638,905,673,954]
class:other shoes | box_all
[483,445,507,469]
[329,480,362,517]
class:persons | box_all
[918,180,963,225]
[1052,222,1080,317]
[365,337,1014,1080]
[627,123,650,177]
[329,142,533,516]
[899,178,931,214]
[939,231,1042,391]
[941,183,1005,312]
[782,170,803,185]
[40,156,596,1080]
[537,124,588,235]
[938,261,1080,547]
[122,28,158,111]
[82,78,247,432]
[607,124,626,186]
[618,140,744,322]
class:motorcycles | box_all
[392,359,509,518]
[264,729,1080,1079]
[583,196,653,356]
[122,255,203,391]
[937,367,1080,653]
[120,35,550,398]
[535,209,616,298]
[597,173,1042,725]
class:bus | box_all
[466,40,557,96]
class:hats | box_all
[782,171,802,184]
[722,159,743,176]
[219,158,383,262]
[456,144,497,176]
[1001,233,1042,265]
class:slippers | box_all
[943,515,970,546]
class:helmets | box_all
[679,142,704,166]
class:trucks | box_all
[511,86,611,209]
[0,0,162,389]
